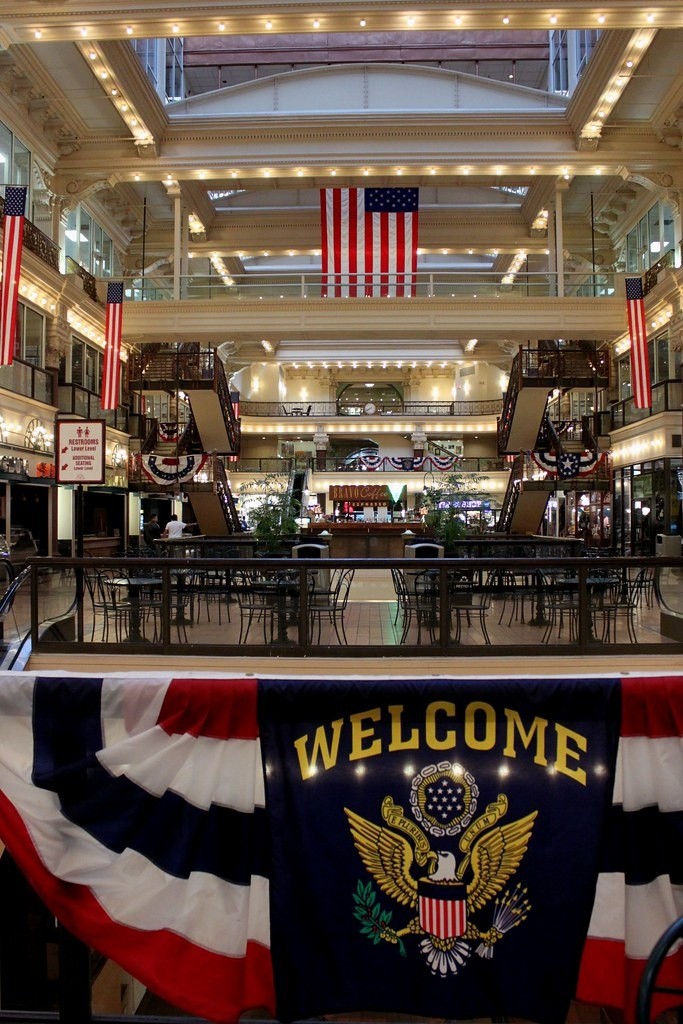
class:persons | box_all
[161,514,200,539]
[142,513,167,550]
[578,503,610,544]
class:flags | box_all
[100,281,125,410]
[226,391,240,462]
[254,679,622,1024]
[0,185,29,367]
[625,276,654,408]
[401,457,414,471]
[556,452,581,479]
[317,186,420,300]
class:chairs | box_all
[1,528,668,649]
